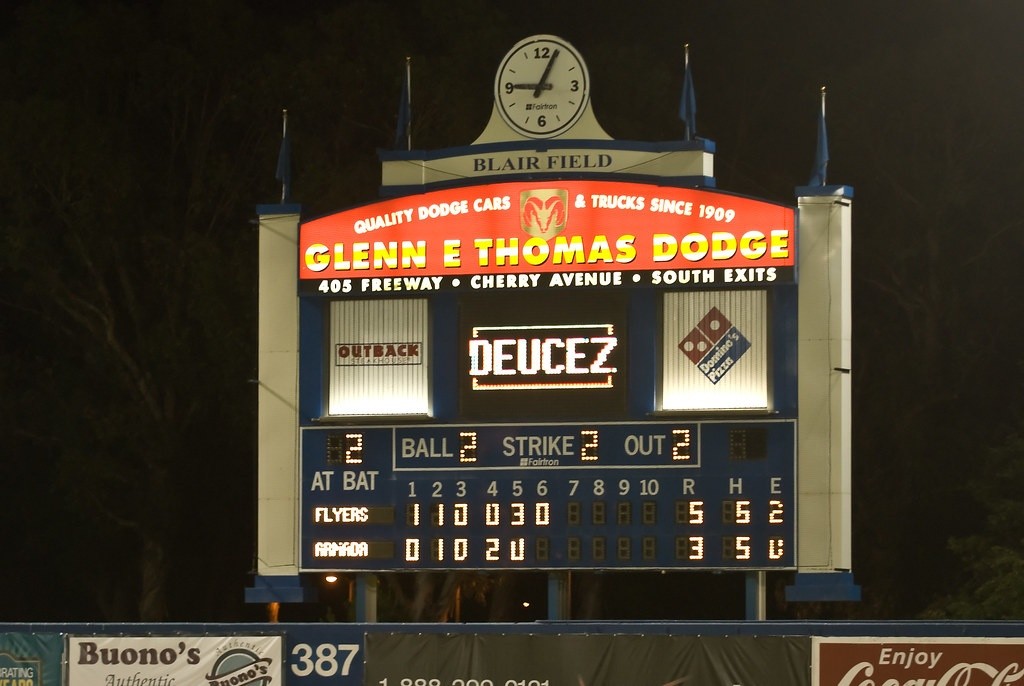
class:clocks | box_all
[495,35,589,139]
